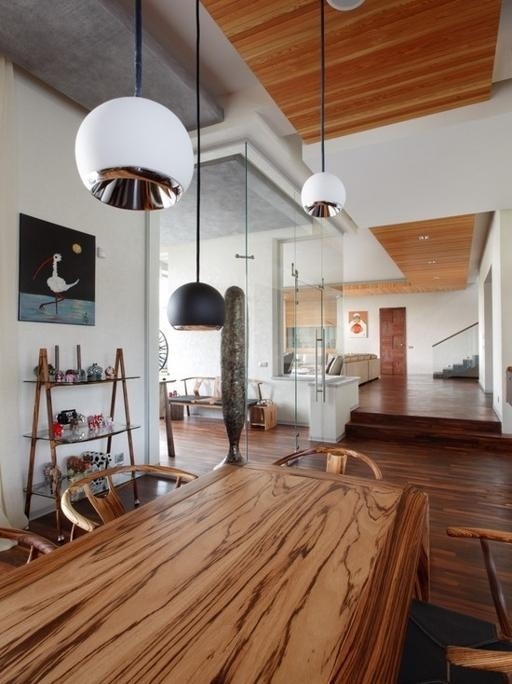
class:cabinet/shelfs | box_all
[22,347,141,543]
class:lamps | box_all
[299,0,346,221]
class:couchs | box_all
[326,354,381,385]
